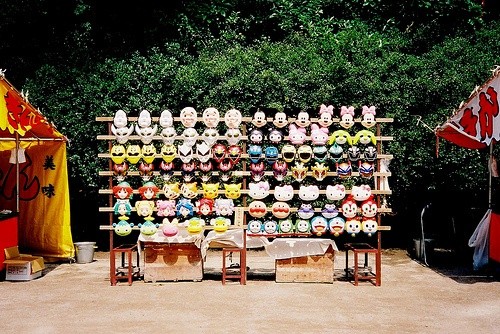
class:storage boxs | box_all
[275,238,334,283]
[3,246,46,280]
[143,243,203,282]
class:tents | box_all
[0,66,75,264]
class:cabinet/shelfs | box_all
[95,117,394,286]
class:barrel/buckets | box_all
[73,242,96,263]
